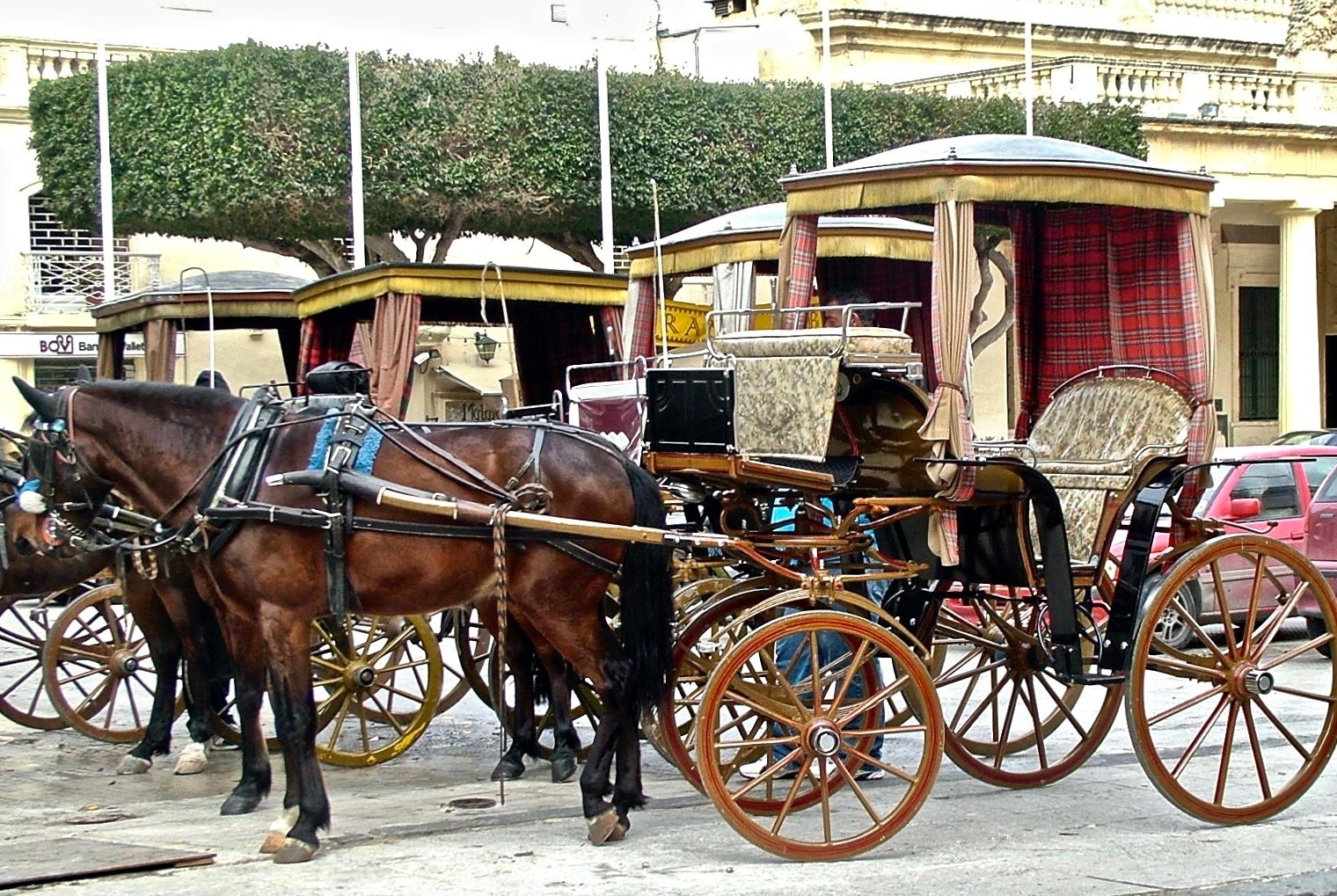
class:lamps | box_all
[475,329,497,364]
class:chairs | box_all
[848,375,943,488]
[1257,485,1319,520]
[1018,364,1193,587]
[706,298,923,462]
[500,390,564,426]
[643,366,865,491]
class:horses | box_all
[2,374,678,863]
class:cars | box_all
[1075,429,1336,654]
[1267,426,1337,449]
[1291,465,1337,660]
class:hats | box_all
[195,370,230,392]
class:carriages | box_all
[0,125,1337,865]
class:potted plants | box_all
[414,328,449,347]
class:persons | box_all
[824,286,928,392]
[740,489,886,779]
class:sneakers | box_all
[206,722,242,750]
[738,753,806,780]
[808,759,885,780]
